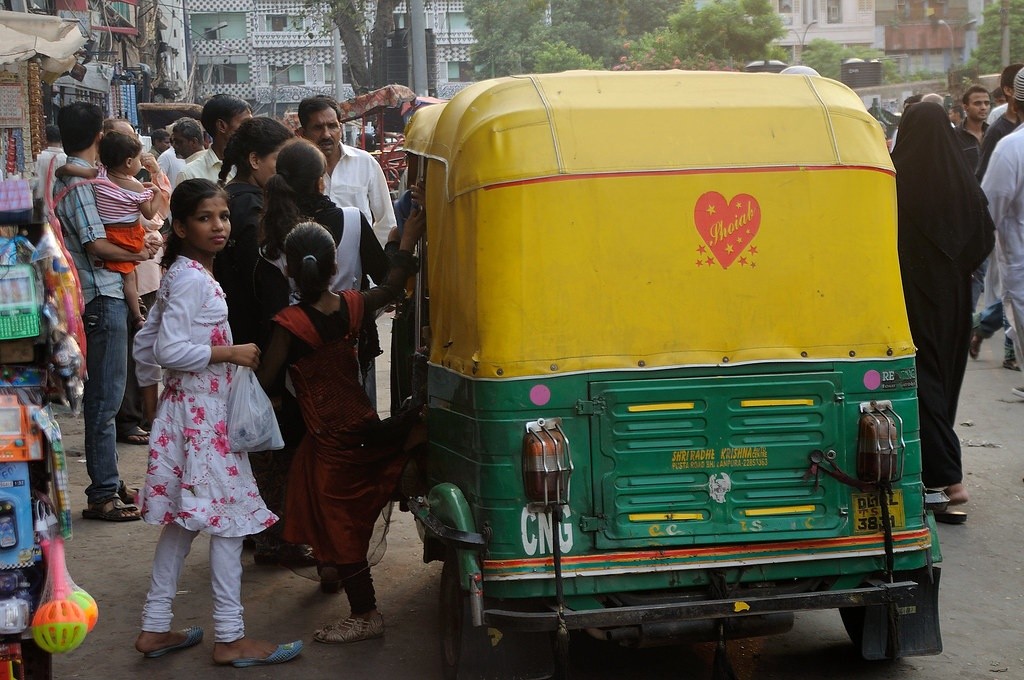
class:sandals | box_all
[81,480,143,522]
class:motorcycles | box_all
[392,69,944,680]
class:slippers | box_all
[935,510,968,525]
[143,625,204,658]
[116,425,151,445]
[229,638,305,668]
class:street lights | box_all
[939,20,954,69]
[786,21,818,65]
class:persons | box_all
[33,94,397,522]
[887,64,1024,397]
[132,178,303,662]
[889,102,997,525]
[255,211,426,644]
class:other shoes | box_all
[309,610,386,645]
[1002,360,1021,372]
[1011,385,1024,399]
[969,327,982,359]
